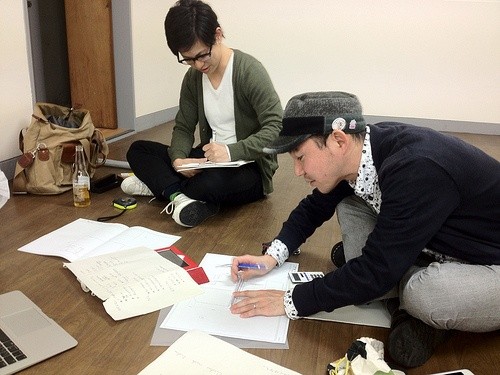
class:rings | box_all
[253,304,256,309]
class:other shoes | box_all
[384,313,438,370]
[331,241,346,267]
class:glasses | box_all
[176,40,213,65]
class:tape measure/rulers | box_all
[112,197,138,210]
[229,278,244,307]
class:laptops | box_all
[0,290,78,375]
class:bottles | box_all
[73,146,91,207]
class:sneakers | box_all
[120,175,157,204]
[159,193,220,228]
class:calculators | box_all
[288,272,325,284]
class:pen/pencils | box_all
[228,264,269,270]
[212,129,217,142]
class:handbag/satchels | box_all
[12,102,109,194]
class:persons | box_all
[121,0,284,230]
[230,90,500,368]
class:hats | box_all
[263,91,366,154]
